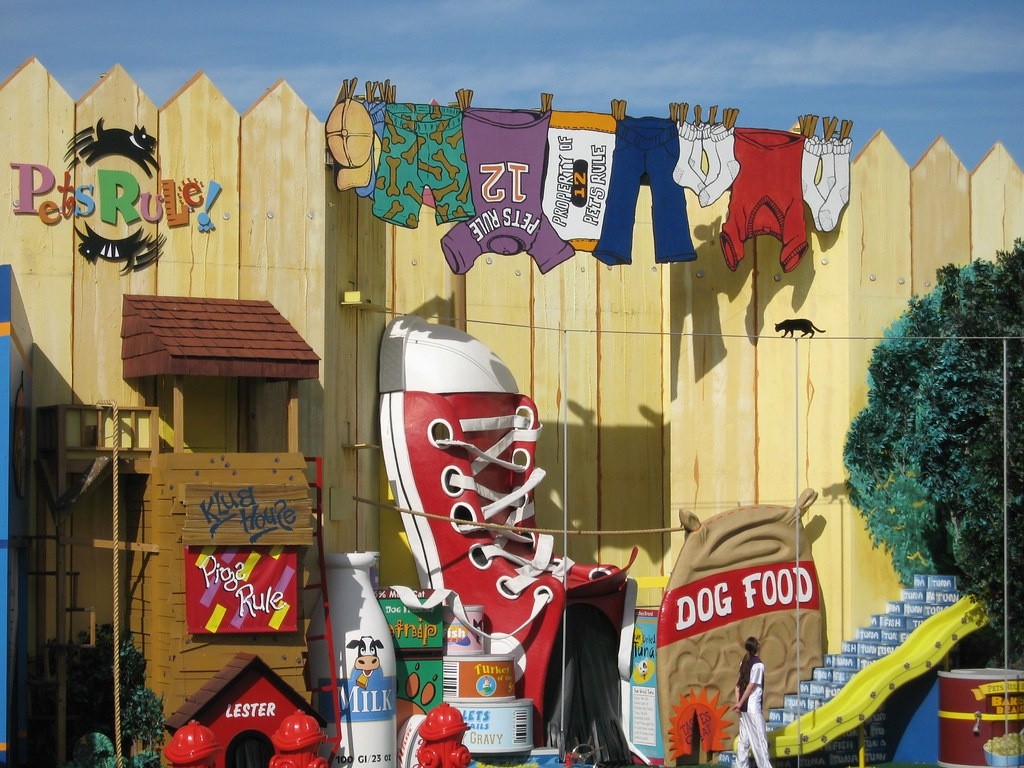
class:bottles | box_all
[306,552,395,768]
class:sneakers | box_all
[377,313,638,750]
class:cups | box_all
[938,667,1024,768]
[983,738,1023,767]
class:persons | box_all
[732,636,774,768]
